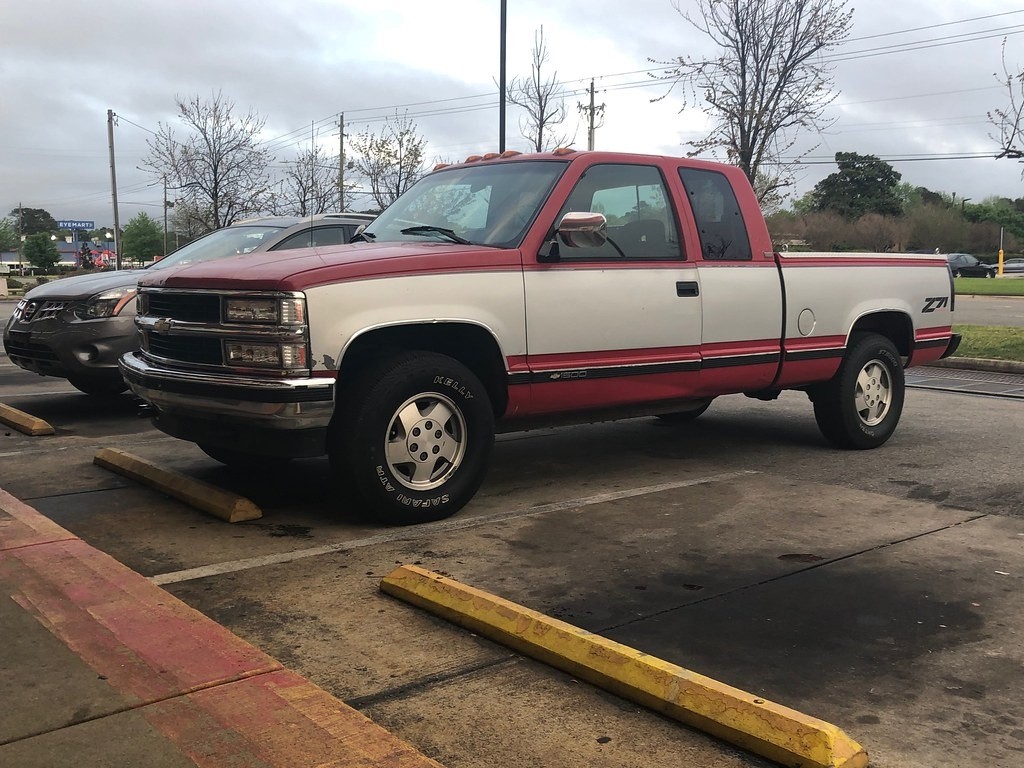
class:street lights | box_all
[105,232,112,263]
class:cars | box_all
[989,258,1024,274]
[946,253,995,278]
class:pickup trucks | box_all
[118,148,962,530]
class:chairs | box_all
[615,220,667,258]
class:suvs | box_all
[2,210,440,420]
